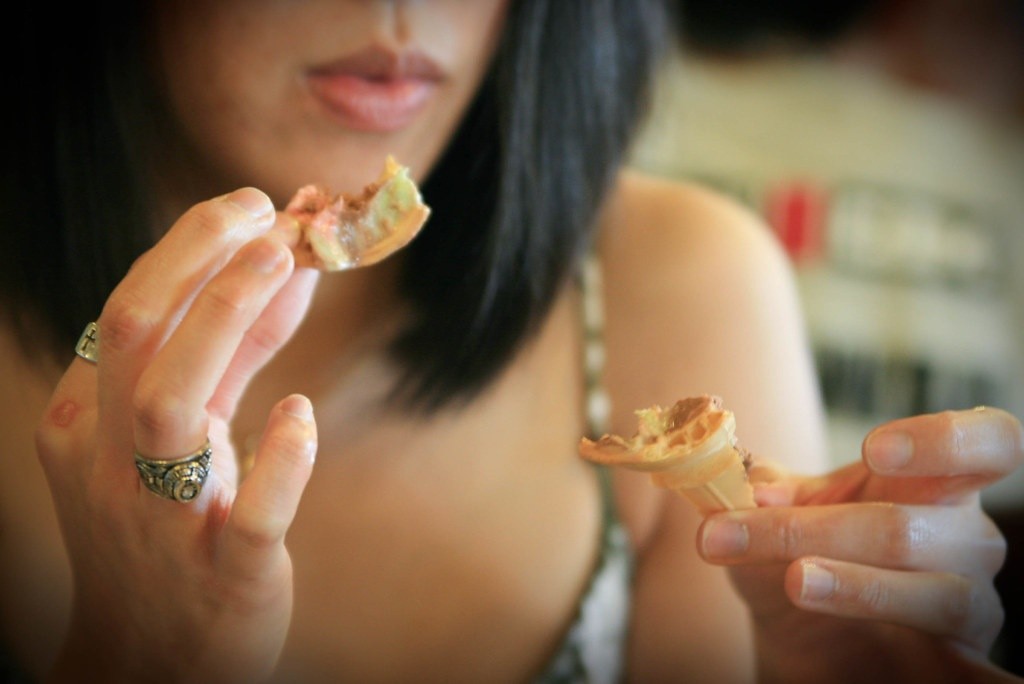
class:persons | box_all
[0,0,1024,684]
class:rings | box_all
[75,323,101,361]
[134,439,211,504]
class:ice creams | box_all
[274,154,432,272]
[579,394,759,519]
